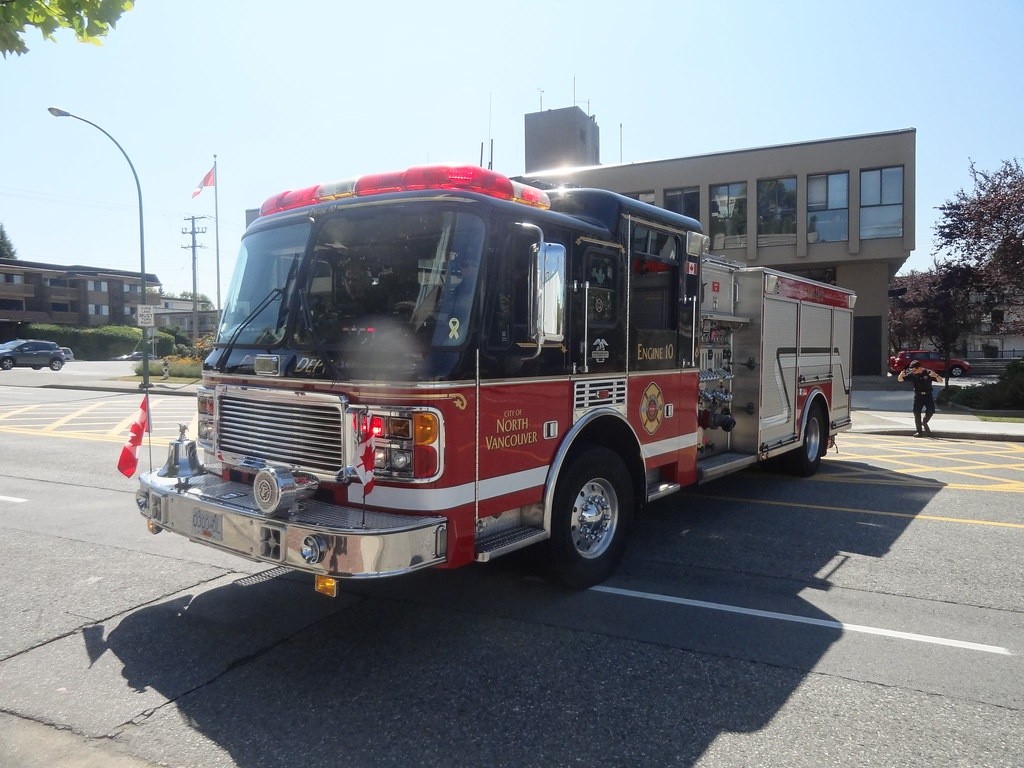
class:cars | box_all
[109,351,158,360]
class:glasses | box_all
[912,368,918,372]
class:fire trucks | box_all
[132,162,862,592]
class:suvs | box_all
[0,339,66,371]
[890,350,972,377]
[59,347,75,360]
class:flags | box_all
[192,166,215,197]
[118,394,153,477]
[353,414,378,496]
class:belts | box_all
[915,391,933,395]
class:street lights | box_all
[48,106,157,389]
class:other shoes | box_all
[923,422,930,431]
[913,432,922,437]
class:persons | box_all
[898,360,942,437]
[337,254,408,319]
[447,258,501,348]
